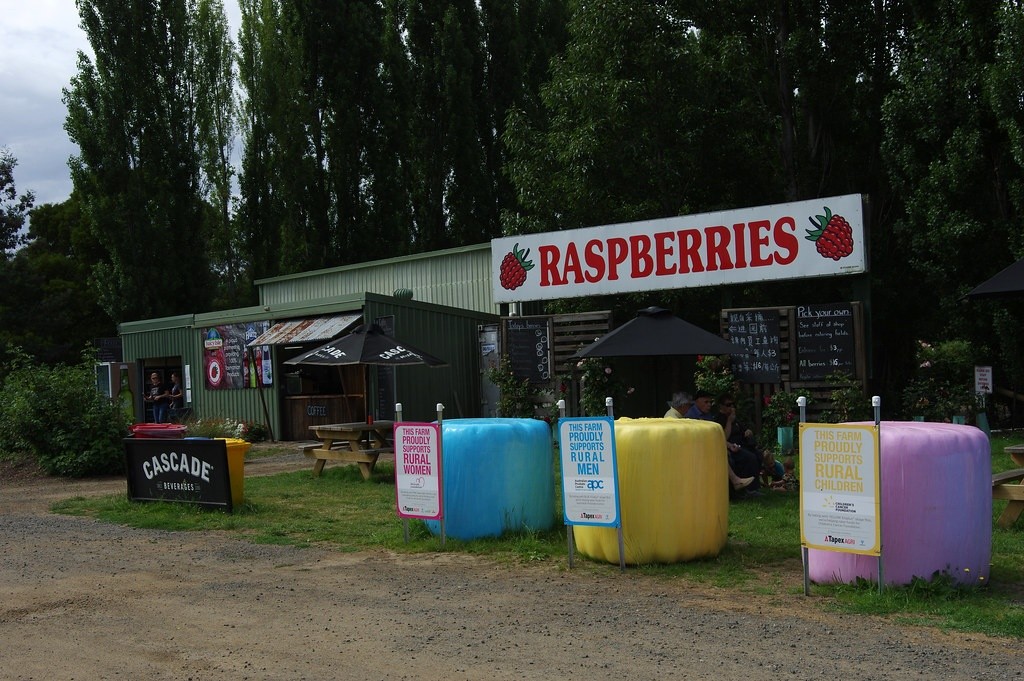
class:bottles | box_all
[204,320,273,389]
[116,364,135,424]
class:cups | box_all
[368,412,373,425]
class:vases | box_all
[778,427,794,453]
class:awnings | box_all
[246,312,362,348]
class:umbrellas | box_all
[565,307,749,418]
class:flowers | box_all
[761,390,797,425]
[544,381,567,422]
[695,356,718,365]
[576,334,610,412]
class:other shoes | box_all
[733,476,755,490]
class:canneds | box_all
[367,413,373,423]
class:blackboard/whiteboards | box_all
[727,311,782,384]
[795,302,855,380]
[501,315,555,384]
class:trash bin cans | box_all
[184,436,251,506]
[130,422,187,438]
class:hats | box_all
[695,390,717,400]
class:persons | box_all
[662,390,799,495]
[281,323,450,449]
[145,373,169,423]
[169,373,183,409]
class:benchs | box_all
[364,446,394,453]
[992,469,1024,487]
[297,440,374,450]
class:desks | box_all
[307,419,395,481]
[993,445,1024,528]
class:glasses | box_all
[721,402,735,408]
